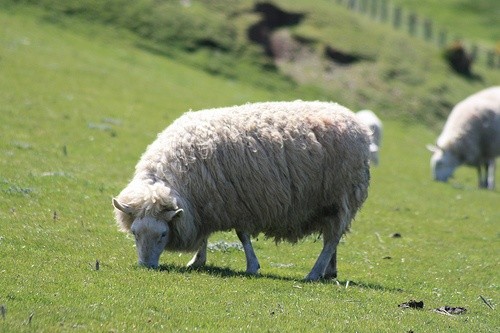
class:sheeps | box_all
[425,86,500,190]
[111,101,382,283]
